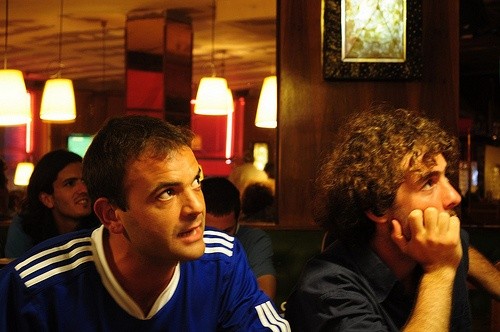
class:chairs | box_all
[258,227,329,319]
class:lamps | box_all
[198,49,234,115]
[0,0,32,126]
[253,75,277,129]
[39,0,77,120]
[194,0,230,114]
[13,161,35,186]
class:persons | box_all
[201,176,276,305]
[1,114,293,332]
[0,150,500,225]
[287,101,499,331]
[5,149,92,258]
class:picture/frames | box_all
[320,0,426,83]
